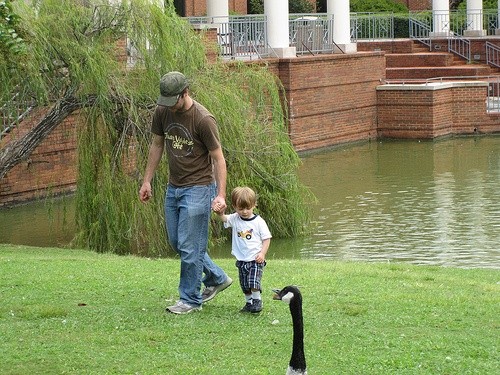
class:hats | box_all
[156,70,188,108]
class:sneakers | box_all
[201,274,233,305]
[166,300,203,315]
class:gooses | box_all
[272,285,309,375]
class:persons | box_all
[139,71,272,314]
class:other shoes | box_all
[239,302,252,313]
[250,298,263,312]
